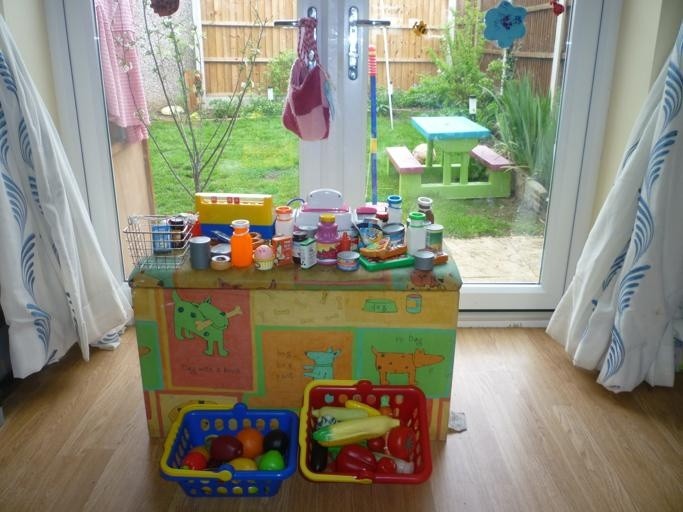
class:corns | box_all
[312,399,401,447]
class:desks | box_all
[409,114,490,198]
[122,230,464,444]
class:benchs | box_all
[386,144,516,200]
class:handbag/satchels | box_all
[281,60,329,140]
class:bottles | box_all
[418,197,434,224]
[406,212,426,257]
[313,212,341,265]
[275,206,294,236]
[340,232,351,251]
[387,195,402,223]
[229,219,253,267]
[293,230,309,263]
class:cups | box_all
[190,236,211,269]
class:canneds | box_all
[170,220,184,248]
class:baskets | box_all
[124,214,194,270]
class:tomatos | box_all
[336,437,397,476]
